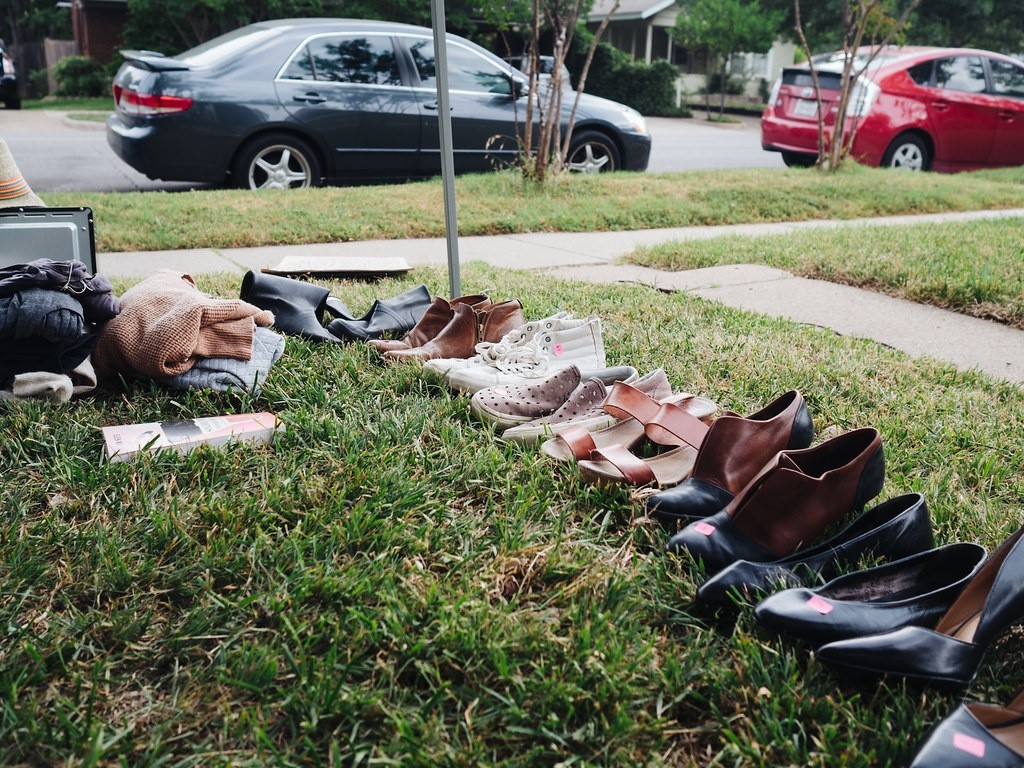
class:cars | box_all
[761,44,1024,169]
[107,17,651,189]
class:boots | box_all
[325,284,432,344]
[240,271,342,349]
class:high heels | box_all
[641,388,813,527]
[664,427,885,576]
[910,689,1024,768]
[818,524,1024,685]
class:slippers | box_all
[577,403,716,490]
[539,380,717,467]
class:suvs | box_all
[0,38,21,109]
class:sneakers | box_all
[421,311,575,383]
[470,364,639,428]
[501,366,672,448]
[445,315,606,394]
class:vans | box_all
[504,55,573,90]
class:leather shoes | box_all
[695,492,934,632]
[380,299,525,366]
[752,542,988,651]
[366,294,491,354]
